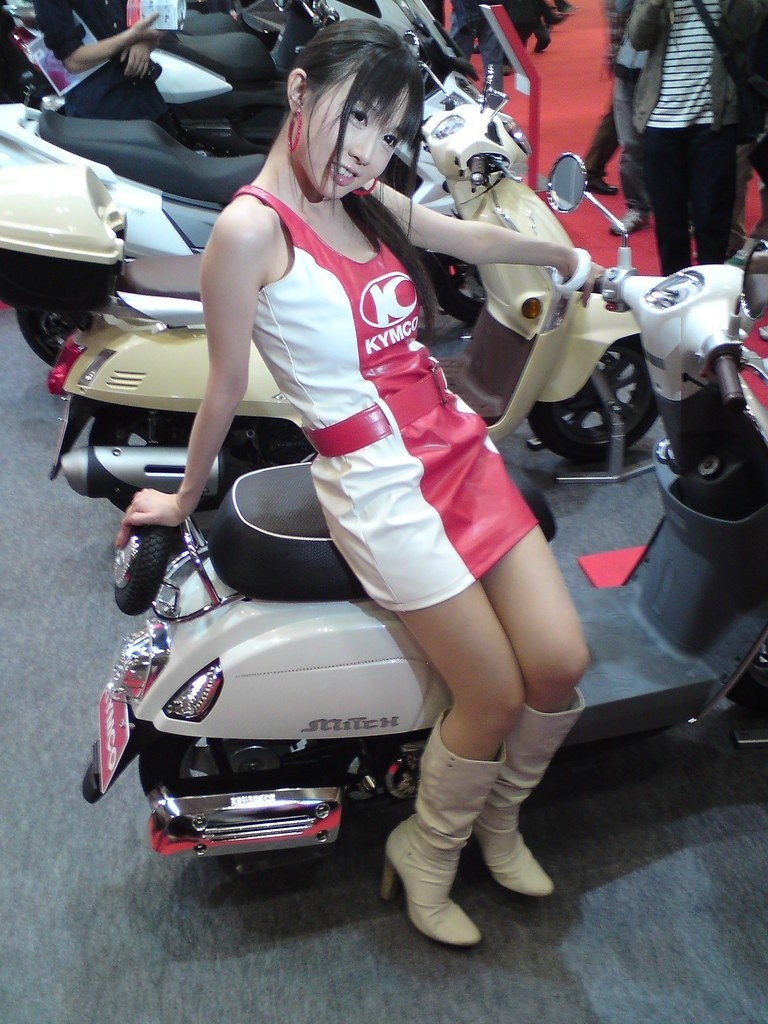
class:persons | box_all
[31,0,177,139]
[424,0,578,113]
[583,0,768,277]
[114,17,607,945]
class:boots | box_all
[382,708,507,947]
[472,686,585,897]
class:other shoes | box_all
[555,3,576,13]
[534,34,550,52]
[502,63,511,75]
[544,13,567,26]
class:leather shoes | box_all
[585,176,618,195]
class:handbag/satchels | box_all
[737,78,757,144]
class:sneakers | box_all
[609,209,650,235]
[723,239,767,268]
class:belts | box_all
[302,366,447,458]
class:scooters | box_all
[0,0,485,367]
[0,31,660,513]
[83,152,768,894]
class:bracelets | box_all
[552,248,591,292]
